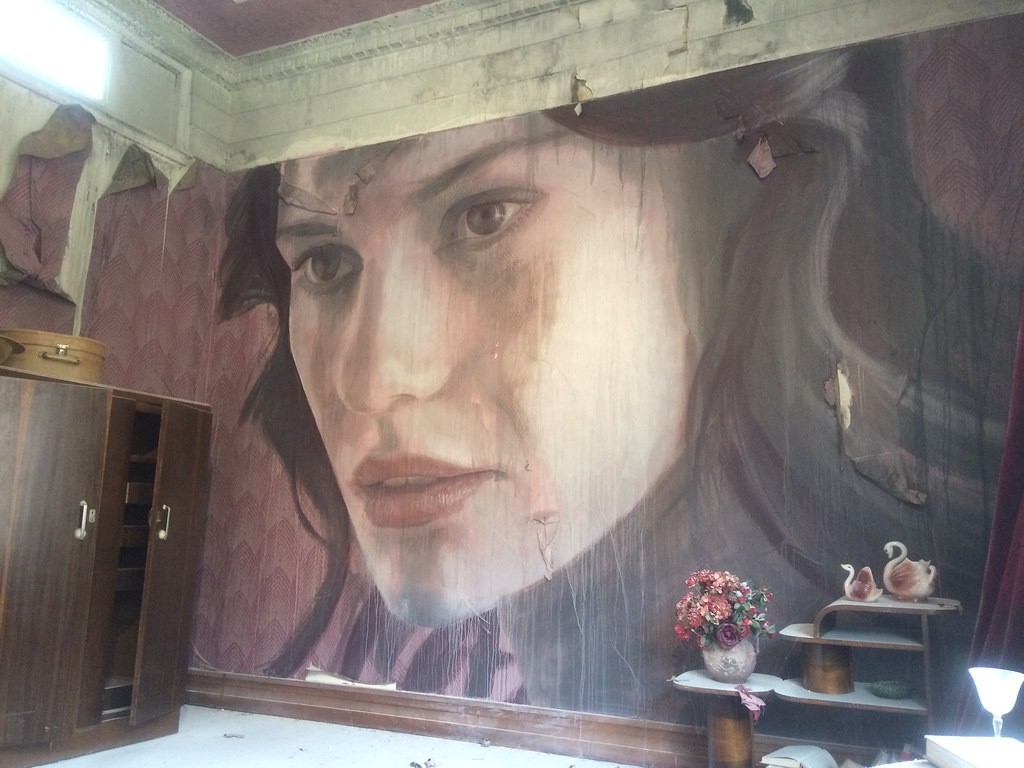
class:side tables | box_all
[672,669,783,768]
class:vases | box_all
[702,636,758,684]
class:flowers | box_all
[675,568,776,655]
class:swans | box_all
[841,563,884,603]
[882,540,937,603]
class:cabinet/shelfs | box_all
[0,362,216,768]
[923,736,1024,768]
[773,590,963,734]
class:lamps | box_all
[967,666,1024,739]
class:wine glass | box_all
[968,667,1024,747]
